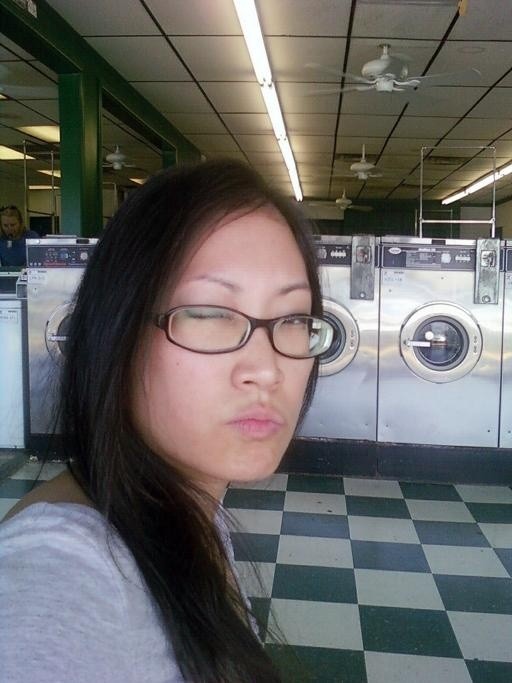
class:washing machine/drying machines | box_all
[379,238,506,448]
[2,266,29,450]
[294,233,382,448]
[25,235,100,436]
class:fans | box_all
[102,143,138,170]
[302,43,471,213]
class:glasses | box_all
[134,303,342,360]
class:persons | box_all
[0,156,338,681]
[0,206,43,290]
